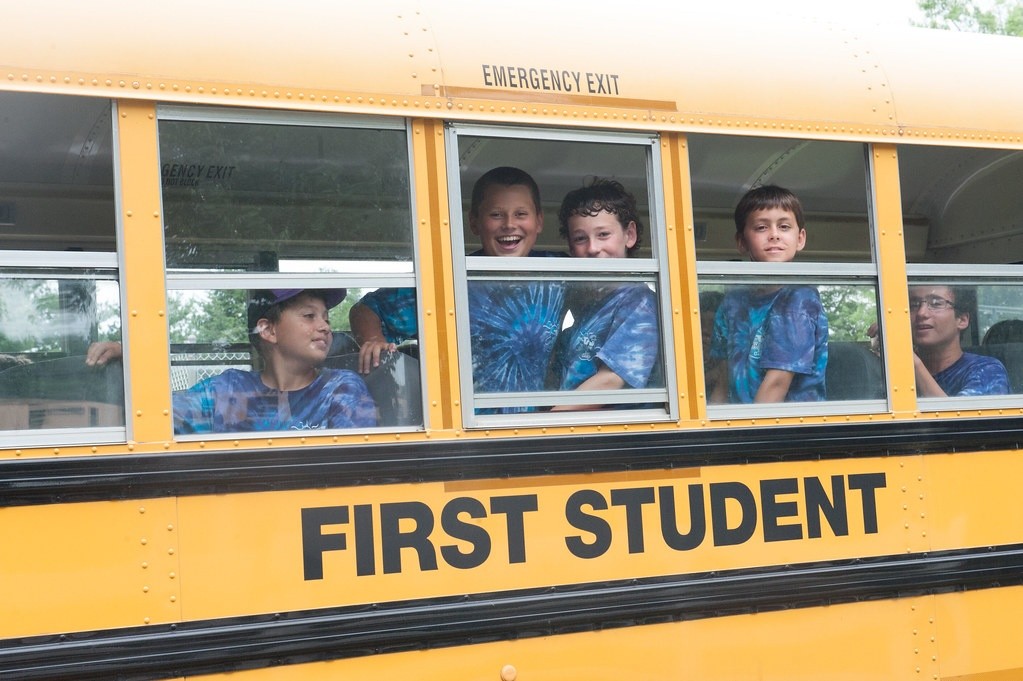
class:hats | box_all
[247,289,347,331]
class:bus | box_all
[1,2,1023,681]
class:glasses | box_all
[911,296,958,313]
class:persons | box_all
[548,181,667,410]
[349,165,586,415]
[866,276,1011,397]
[701,183,828,405]
[86,288,379,435]
[0,354,31,371]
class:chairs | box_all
[0,318,1023,438]
